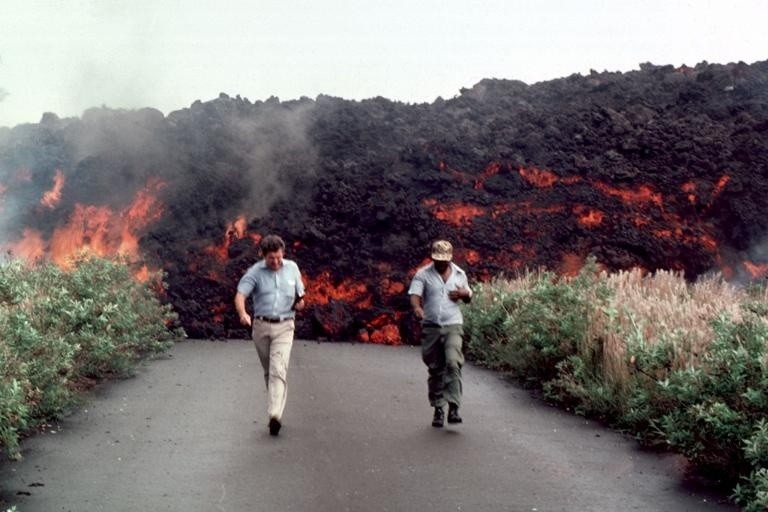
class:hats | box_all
[431,240,453,263]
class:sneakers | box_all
[432,409,445,426]
[447,406,462,423]
[269,417,281,436]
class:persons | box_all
[407,238,474,429]
[233,234,306,436]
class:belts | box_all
[256,314,293,324]
